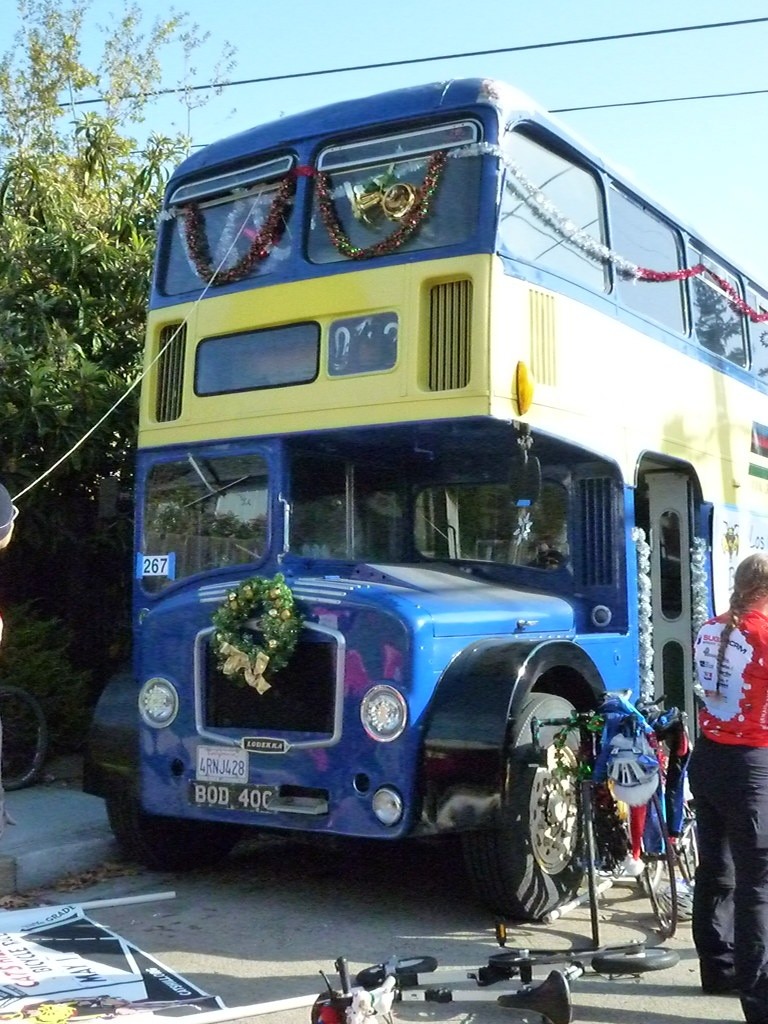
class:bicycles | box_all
[533,692,698,948]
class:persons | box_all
[687,554,768,1024]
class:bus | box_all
[85,77,767,922]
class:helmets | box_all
[607,755,661,787]
[657,890,693,921]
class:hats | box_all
[612,773,659,875]
